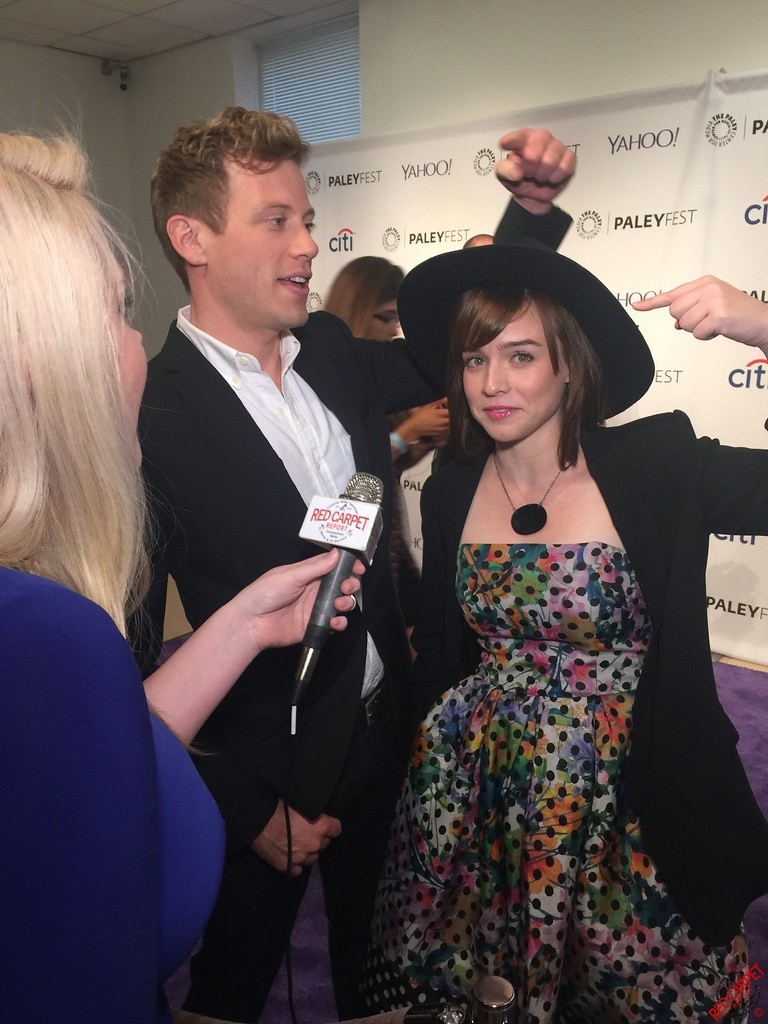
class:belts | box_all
[357,691,389,726]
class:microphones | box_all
[292,472,385,684]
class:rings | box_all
[350,595,356,610]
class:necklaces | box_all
[493,453,561,535]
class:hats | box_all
[397,237,656,420]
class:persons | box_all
[357,244,768,1023]
[0,129,367,1023]
[136,107,578,1023]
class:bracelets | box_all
[389,432,408,455]
